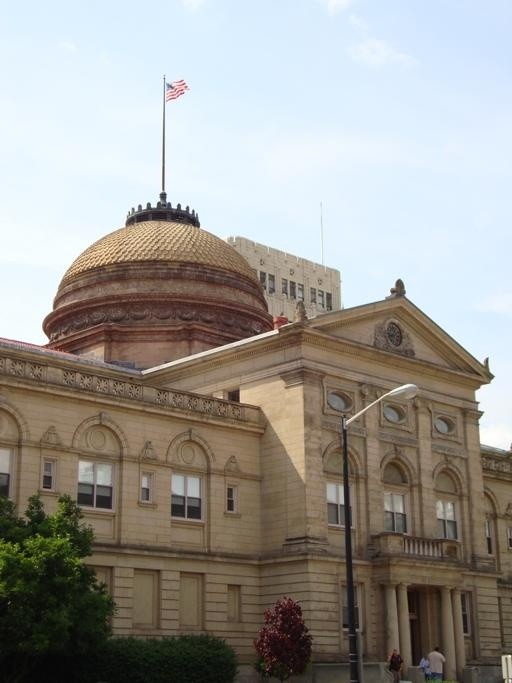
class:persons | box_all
[427,647,446,682]
[420,652,431,682]
[389,649,404,683]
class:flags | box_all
[163,79,191,102]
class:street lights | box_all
[339,380,420,683]
